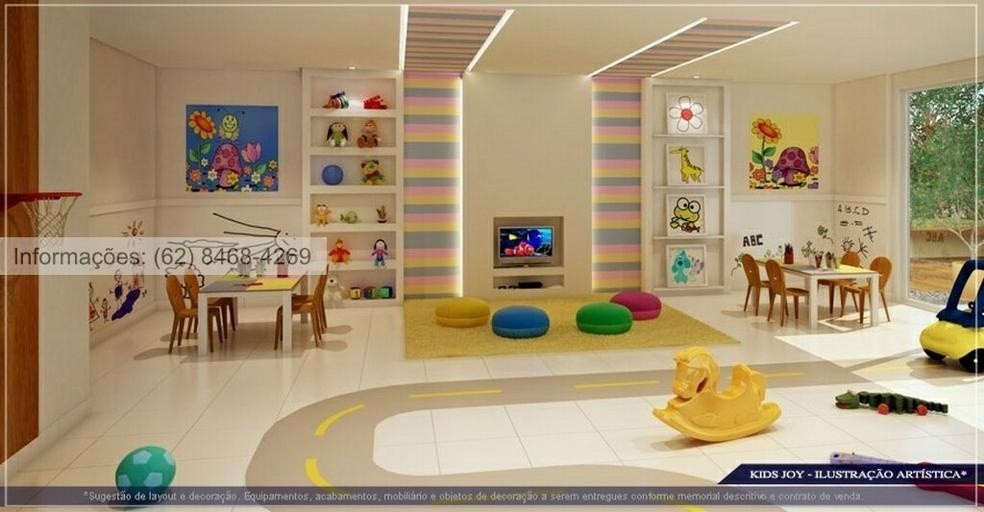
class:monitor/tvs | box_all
[498,225,556,268]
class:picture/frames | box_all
[665,193,706,237]
[666,144,708,185]
[665,244,706,288]
[666,92,707,136]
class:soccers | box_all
[323,165,342,185]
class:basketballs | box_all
[116,447,175,503]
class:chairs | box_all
[741,244,892,329]
[184,267,235,338]
[166,275,223,352]
[274,262,330,348]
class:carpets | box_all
[402,296,741,361]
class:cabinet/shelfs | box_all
[302,67,404,308]
[652,75,728,297]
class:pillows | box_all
[491,304,550,338]
[610,292,661,320]
[576,302,633,334]
[436,297,490,328]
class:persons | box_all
[312,89,393,300]
